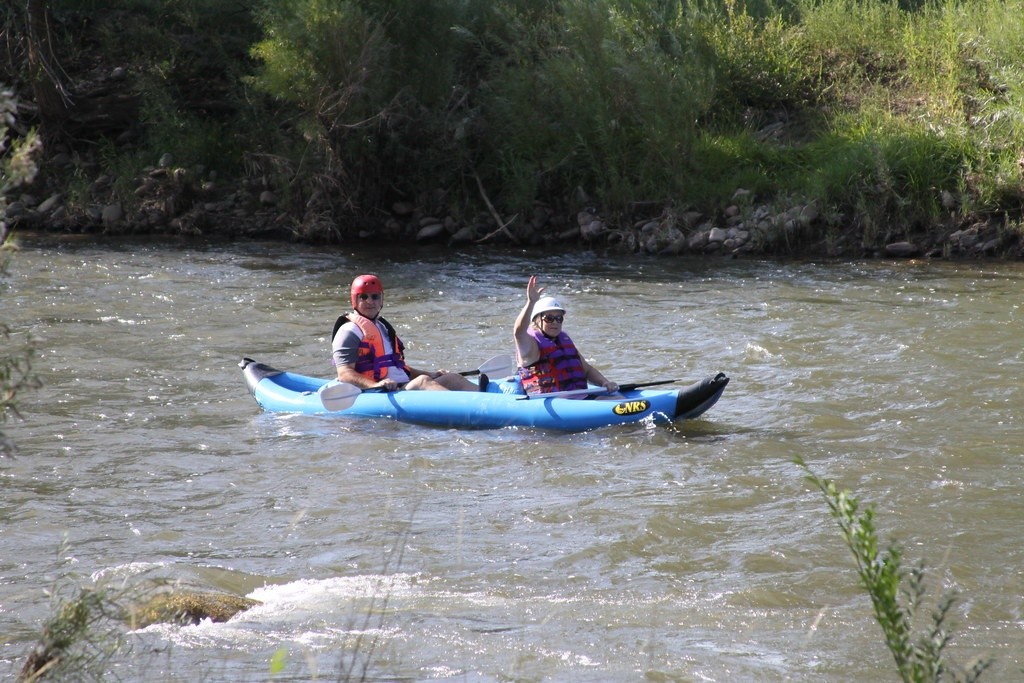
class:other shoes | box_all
[479,374,489,391]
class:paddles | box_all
[515,377,683,402]
[319,352,512,413]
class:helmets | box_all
[531,297,566,322]
[351,275,383,310]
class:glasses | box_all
[541,314,564,323]
[357,294,380,300]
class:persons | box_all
[332,275,489,391]
[513,276,626,401]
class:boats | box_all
[235,355,731,438]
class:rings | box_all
[615,385,617,388]
